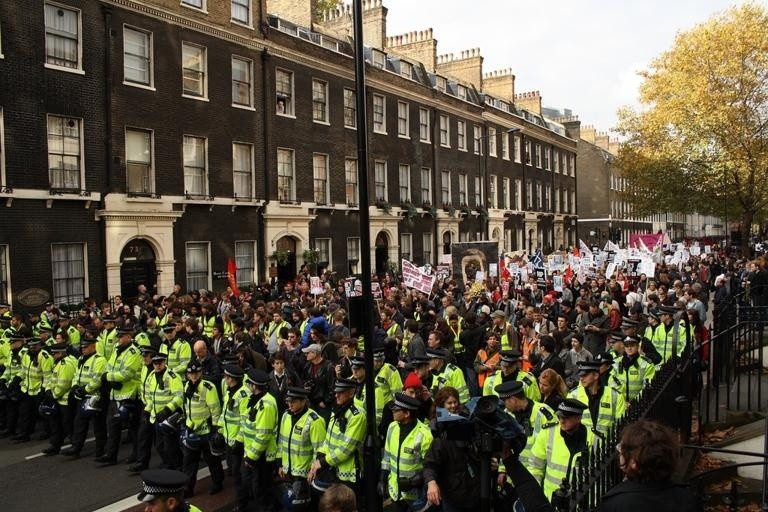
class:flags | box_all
[227,259,242,300]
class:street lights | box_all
[472,126,522,242]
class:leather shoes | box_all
[129,462,149,472]
[41,446,60,455]
[61,446,82,456]
[10,432,31,442]
[94,447,104,457]
[125,457,135,463]
[94,453,118,465]
[208,484,224,496]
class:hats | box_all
[494,381,523,399]
[389,392,420,411]
[115,328,134,337]
[223,365,243,378]
[102,315,116,322]
[373,348,385,360]
[301,344,321,354]
[594,352,615,364]
[186,359,202,373]
[621,335,641,345]
[649,309,658,321]
[244,369,268,386]
[56,315,70,322]
[38,326,53,333]
[499,350,521,367]
[348,356,365,369]
[25,338,41,346]
[620,316,639,328]
[576,361,602,377]
[656,307,674,315]
[51,344,66,352]
[79,338,96,348]
[490,310,506,319]
[139,345,156,355]
[0,302,11,307]
[410,357,429,368]
[333,378,356,392]
[556,398,588,418]
[162,323,176,332]
[402,371,422,390]
[608,332,624,343]
[599,295,612,301]
[424,348,445,359]
[44,301,53,306]
[285,388,307,401]
[8,335,24,343]
[150,353,168,363]
[136,468,190,503]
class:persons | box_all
[410,355,450,392]
[493,380,558,511]
[378,371,431,458]
[423,429,496,511]
[77,258,363,366]
[59,337,108,460]
[531,335,565,381]
[154,360,225,501]
[57,314,79,344]
[300,343,336,420]
[47,307,59,339]
[307,376,368,497]
[611,334,656,404]
[526,398,603,504]
[495,435,559,512]
[137,468,202,512]
[232,369,279,511]
[335,338,365,381]
[4,314,33,340]
[0,335,30,439]
[29,309,52,342]
[39,300,62,324]
[125,345,159,473]
[373,346,402,426]
[8,338,54,442]
[92,328,140,465]
[216,363,252,507]
[473,332,500,396]
[377,392,434,512]
[430,384,469,431]
[346,355,367,411]
[538,368,565,414]
[267,353,300,419]
[562,334,593,377]
[77,321,93,341]
[425,350,471,406]
[370,232,768,350]
[320,482,358,512]
[593,352,628,407]
[39,342,78,455]
[0,302,12,319]
[144,353,184,473]
[482,350,541,403]
[594,420,685,511]
[274,386,327,512]
[565,360,628,463]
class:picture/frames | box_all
[452,242,500,291]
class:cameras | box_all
[435,395,528,469]
